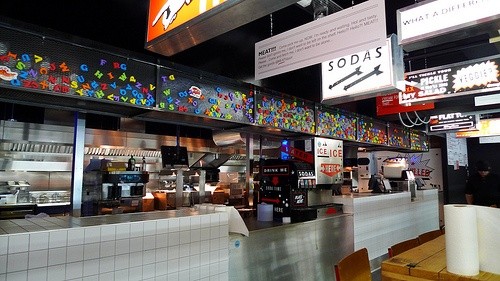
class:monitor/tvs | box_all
[161,145,189,169]
[382,179,392,193]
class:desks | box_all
[381,233,500,281]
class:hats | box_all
[474,160,492,171]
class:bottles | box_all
[129,156,135,171]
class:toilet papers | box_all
[444,203,499,276]
[195,202,250,237]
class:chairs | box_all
[334,248,372,281]
[387,230,443,258]
